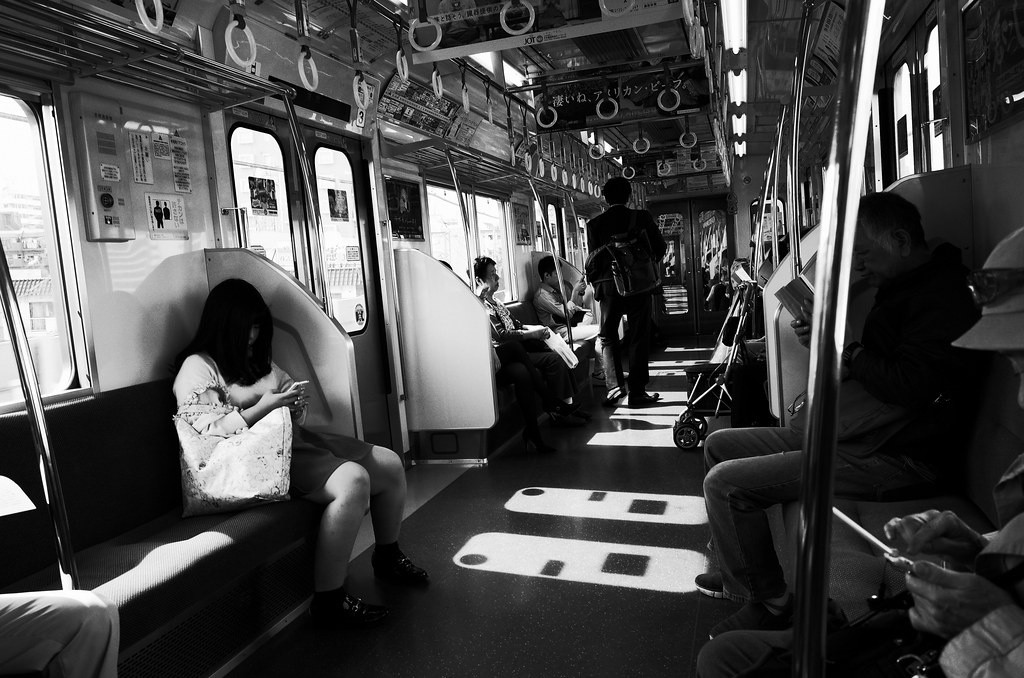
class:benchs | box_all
[761,166,1017,616]
[392,245,604,464]
[21,245,364,677]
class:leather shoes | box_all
[371,549,428,582]
[601,386,627,404]
[629,391,659,407]
[568,402,592,418]
[310,588,389,626]
[561,415,585,426]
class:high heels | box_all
[522,426,557,454]
[541,396,580,421]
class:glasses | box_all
[967,269,1024,307]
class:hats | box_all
[950,226,1024,350]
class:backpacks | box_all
[611,231,661,295]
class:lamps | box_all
[721,0,747,158]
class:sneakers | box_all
[592,372,629,385]
[709,592,794,641]
[695,573,752,602]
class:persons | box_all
[0,589,120,678]
[701,241,788,314]
[695,190,993,641]
[438,260,582,452]
[167,278,427,623]
[587,177,667,406]
[534,256,630,386]
[692,238,1024,678]
[467,256,594,425]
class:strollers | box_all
[673,281,765,450]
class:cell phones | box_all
[285,379,308,398]
[579,275,584,282]
[831,505,916,574]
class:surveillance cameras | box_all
[741,174,751,186]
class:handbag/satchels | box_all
[778,609,945,678]
[722,289,741,347]
[543,326,579,369]
[174,381,292,518]
